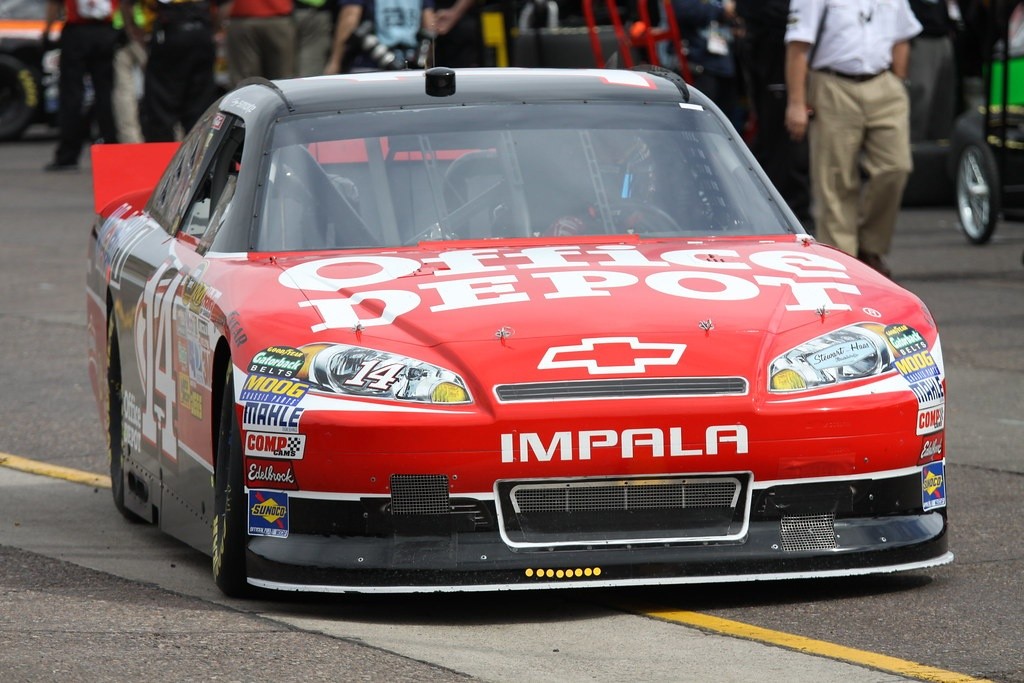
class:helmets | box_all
[575,133,654,224]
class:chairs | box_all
[258,166,363,249]
[444,151,548,236]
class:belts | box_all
[819,67,886,82]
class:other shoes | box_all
[858,250,892,279]
[45,160,78,170]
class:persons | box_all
[112,0,482,145]
[40,0,138,171]
[540,128,660,239]
[671,0,1006,233]
[784,0,924,280]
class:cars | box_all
[2,0,1024,248]
[84,61,956,604]
